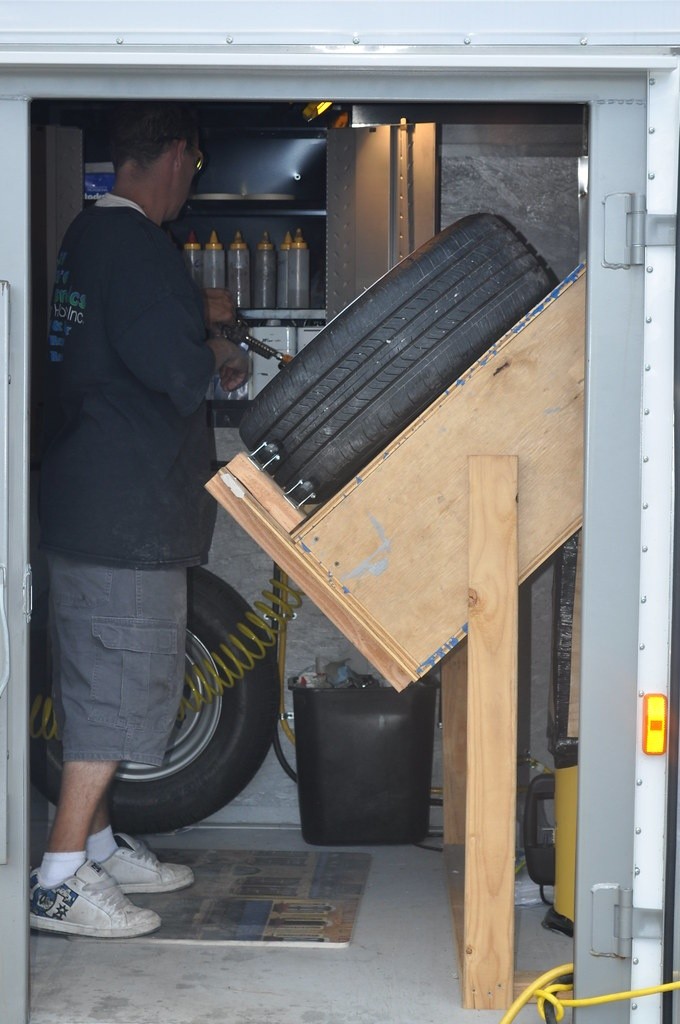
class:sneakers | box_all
[30,860,161,937]
[100,832,193,894]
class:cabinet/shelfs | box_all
[81,122,328,468]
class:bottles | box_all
[183,228,201,284]
[203,231,224,288]
[276,231,290,309]
[225,231,249,310]
[289,229,310,310]
[255,232,275,308]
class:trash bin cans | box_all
[288,676,441,846]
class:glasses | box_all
[185,144,203,173]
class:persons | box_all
[27,102,252,938]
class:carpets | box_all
[66,848,374,947]
